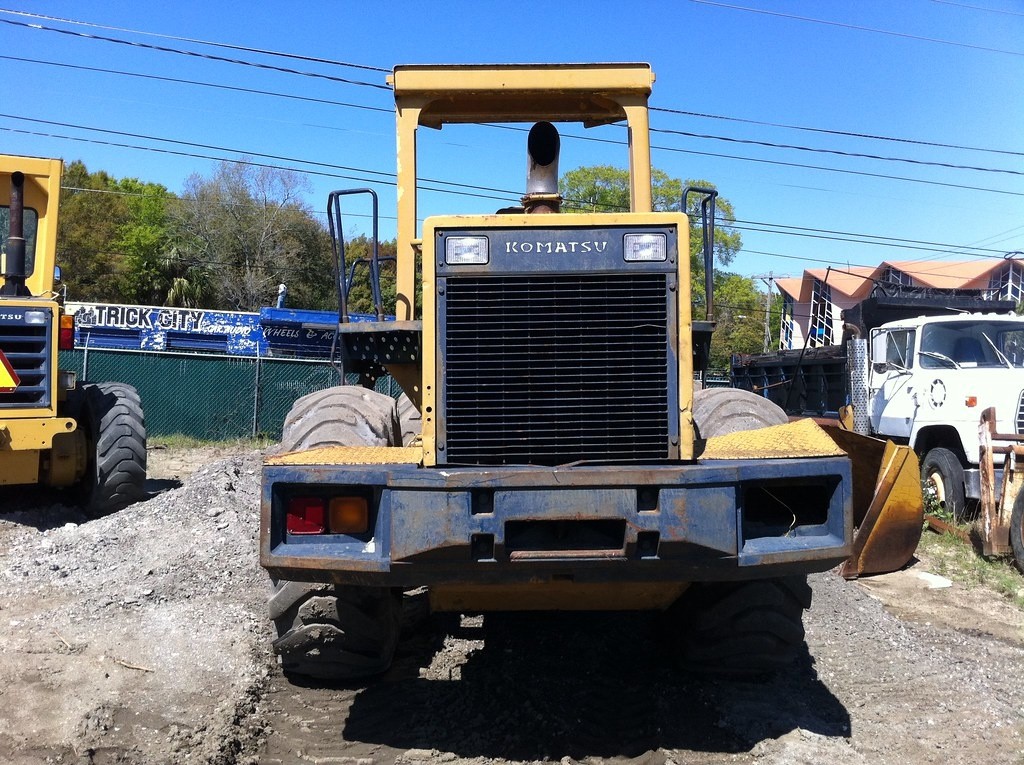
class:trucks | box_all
[728,298,1024,523]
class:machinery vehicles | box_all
[258,63,853,681]
[0,153,149,514]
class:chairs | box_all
[953,336,987,365]
